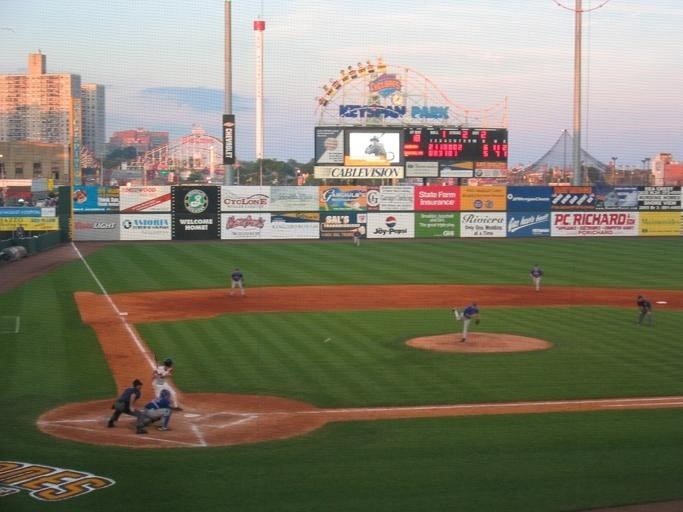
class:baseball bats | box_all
[154,351,158,369]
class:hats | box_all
[133,380,142,385]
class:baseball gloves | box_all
[474,317,481,323]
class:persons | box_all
[106,379,148,433]
[451,302,481,342]
[229,267,245,296]
[364,136,385,158]
[143,388,174,430]
[636,295,654,327]
[16,223,24,238]
[529,264,545,292]
[150,358,183,411]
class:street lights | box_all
[608,156,651,187]
[17,199,25,204]
[0,154,5,207]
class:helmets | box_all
[161,390,170,399]
[164,359,171,366]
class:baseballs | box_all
[324,337,331,343]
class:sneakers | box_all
[108,423,167,433]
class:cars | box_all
[86,177,95,182]
[109,180,119,187]
[35,196,59,208]
